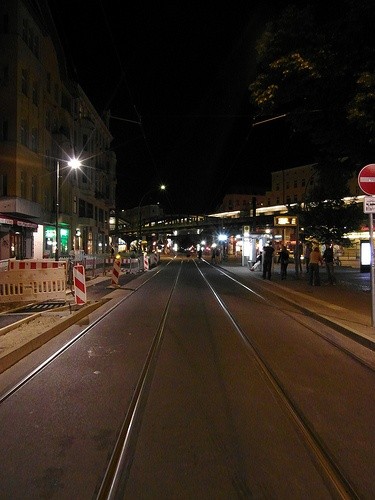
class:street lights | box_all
[54,159,82,261]
[137,184,166,251]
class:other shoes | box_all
[249,267,254,271]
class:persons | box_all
[278,246,289,280]
[250,251,262,271]
[304,242,312,272]
[322,242,337,285]
[295,241,303,272]
[212,250,215,258]
[308,248,323,286]
[261,243,274,280]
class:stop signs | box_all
[357,164,375,196]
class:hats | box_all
[268,241,272,245]
